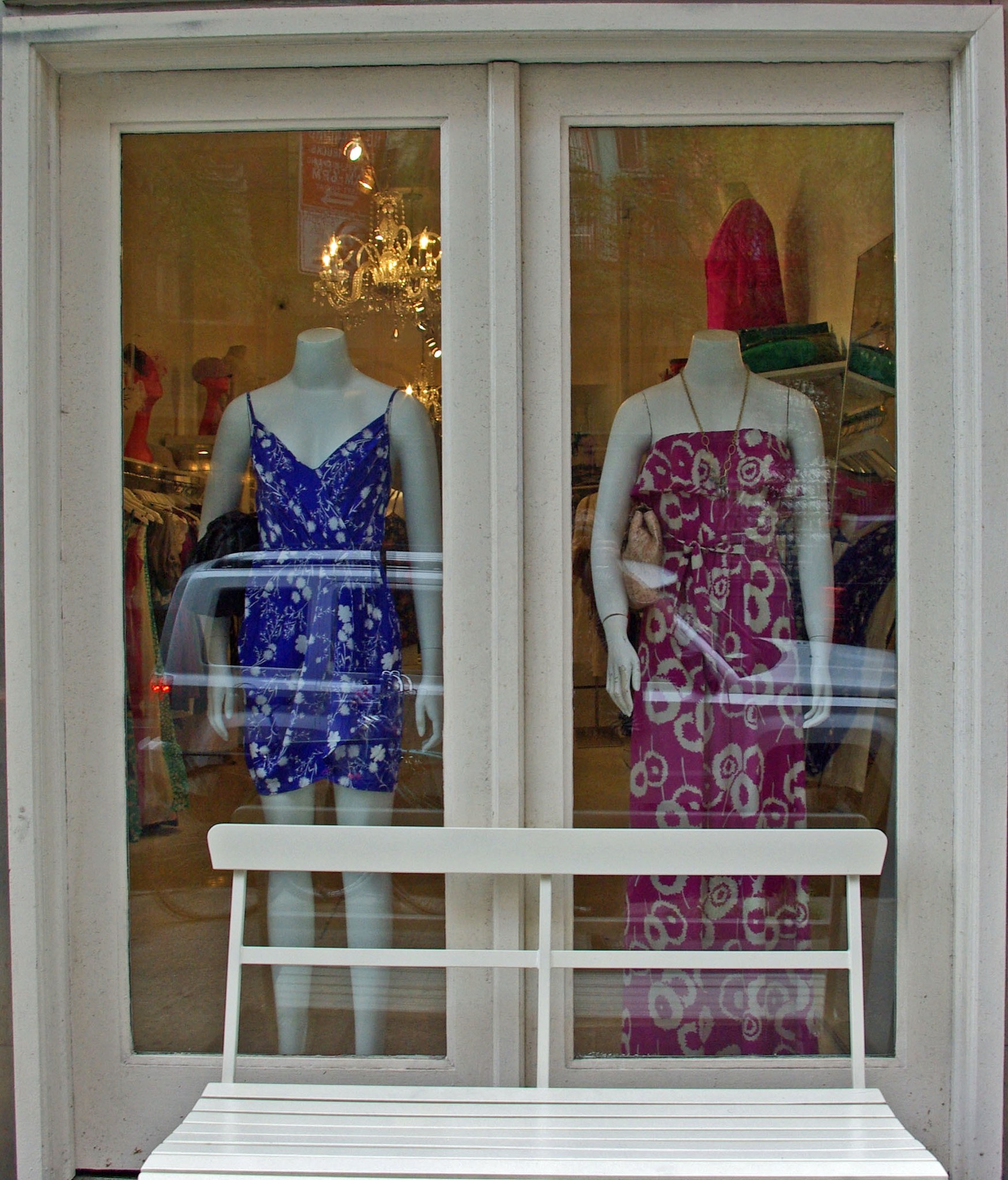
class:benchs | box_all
[131,820,953,1179]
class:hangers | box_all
[122,471,211,530]
[820,405,898,482]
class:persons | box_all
[590,328,840,1057]
[190,324,448,1060]
[120,330,166,474]
[193,348,246,438]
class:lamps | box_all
[311,133,446,344]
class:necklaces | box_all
[677,365,754,497]
